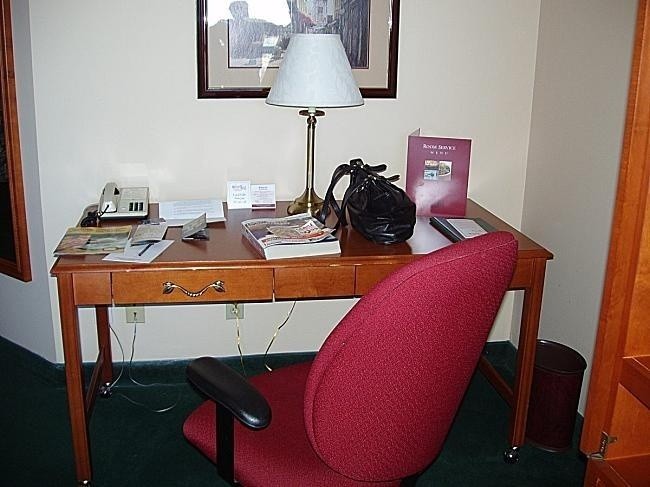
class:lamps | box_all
[265,31,364,216]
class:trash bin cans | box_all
[524,339,588,454]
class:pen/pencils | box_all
[139,244,152,256]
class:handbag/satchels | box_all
[317,158,416,245]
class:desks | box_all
[49,199,552,487]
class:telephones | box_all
[98,182,150,220]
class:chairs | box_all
[182,230,519,486]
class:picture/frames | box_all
[196,0,400,97]
[0,0,32,284]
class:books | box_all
[241,213,342,261]
[429,216,498,243]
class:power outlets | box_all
[225,302,244,320]
